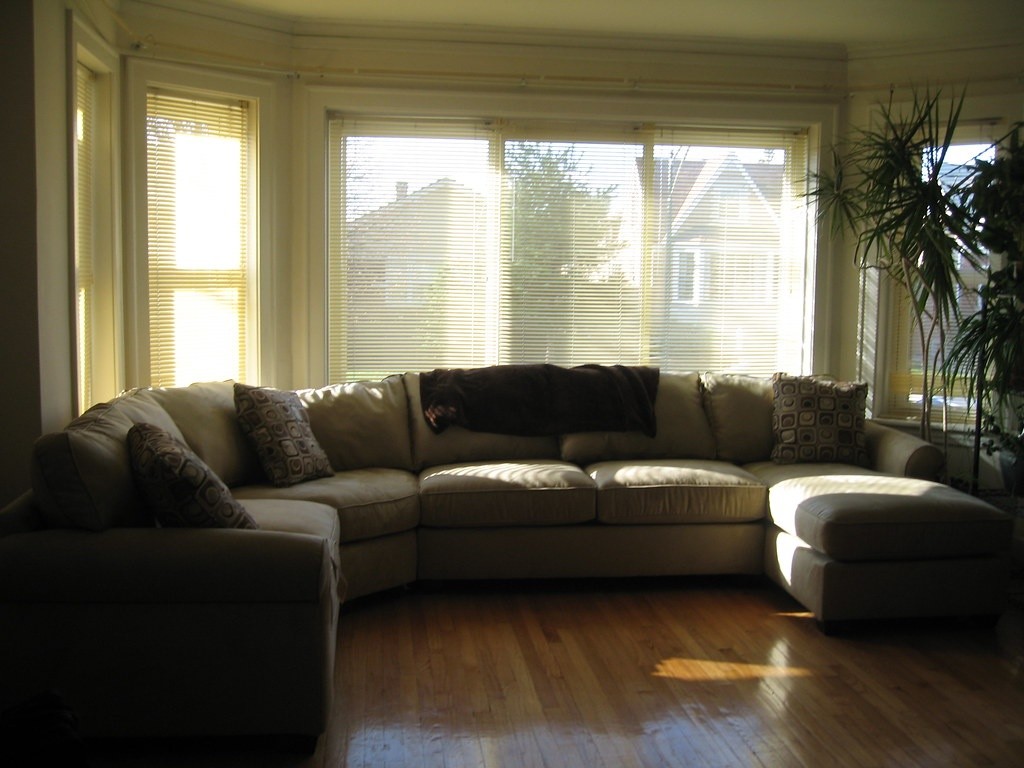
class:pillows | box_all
[235,384,336,487]
[771,373,872,468]
[126,421,257,530]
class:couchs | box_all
[0,361,1022,747]
